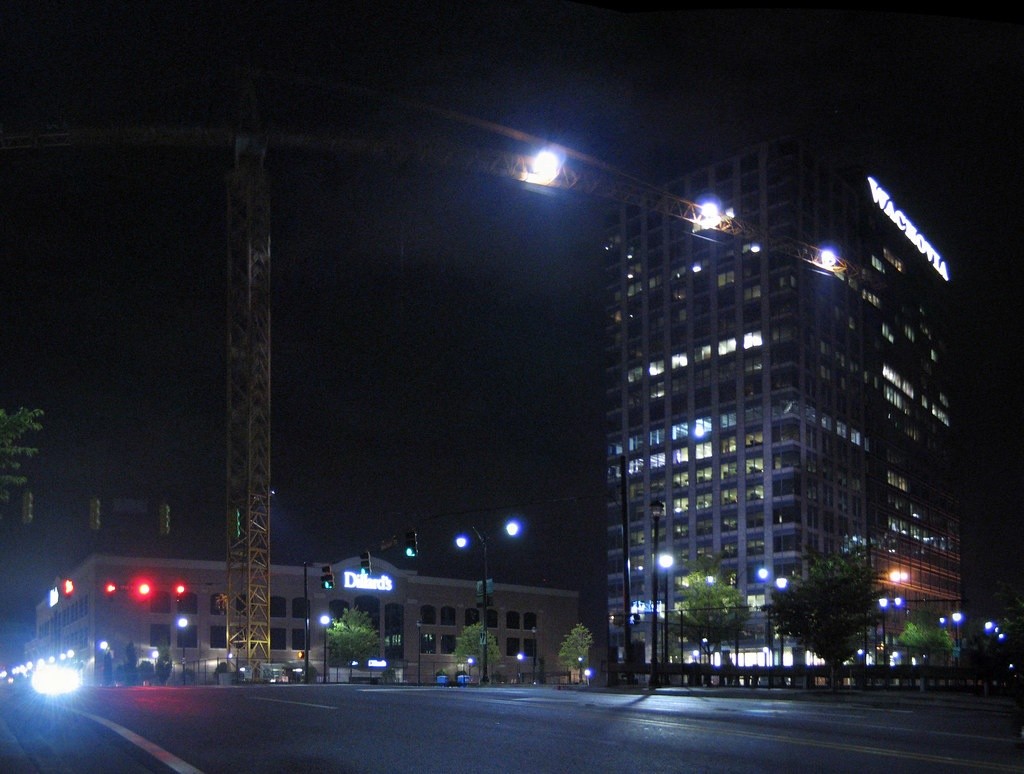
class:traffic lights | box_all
[360,551,372,576]
[407,530,419,558]
[321,566,334,590]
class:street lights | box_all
[179,618,189,686]
[756,567,788,688]
[455,517,524,680]
[649,497,662,692]
[319,615,331,684]
[878,594,891,689]
[416,619,426,686]
[659,554,672,688]
[951,612,962,668]
